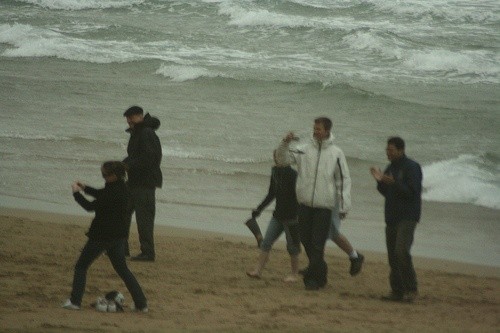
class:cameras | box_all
[293,135,300,141]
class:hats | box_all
[123,105,144,116]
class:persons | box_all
[104,103,162,261]
[370,135,424,301]
[298,208,365,276]
[273,115,352,291]
[247,148,302,282]
[61,159,151,313]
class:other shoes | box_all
[305,274,327,290]
[349,250,364,275]
[384,288,404,301]
[63,299,78,310]
[132,250,153,261]
[95,296,107,312]
[106,299,116,312]
